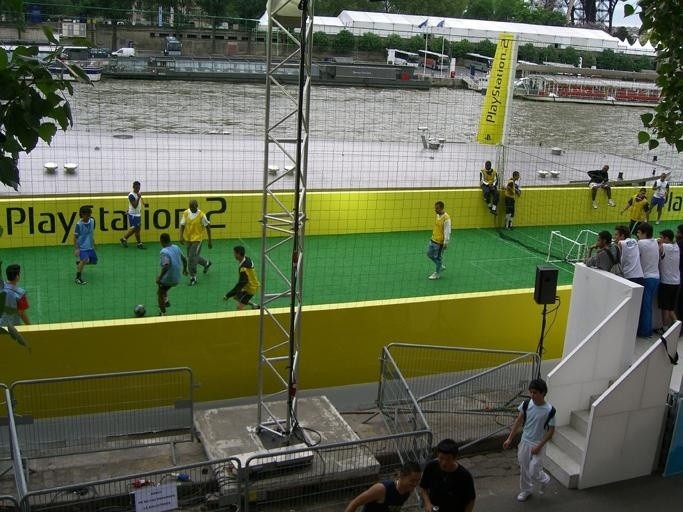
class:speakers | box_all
[533,265,559,305]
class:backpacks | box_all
[602,246,624,279]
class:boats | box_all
[513,74,672,111]
[2,44,102,81]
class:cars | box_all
[90,46,138,59]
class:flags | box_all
[419,20,428,29]
[436,20,443,27]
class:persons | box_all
[418,439,477,512]
[621,189,648,231]
[343,461,422,512]
[427,201,453,280]
[223,245,259,312]
[502,377,556,502]
[155,232,189,317]
[120,180,149,250]
[648,173,671,225]
[479,160,500,216]
[179,199,214,286]
[586,165,616,209]
[587,216,683,338]
[0,264,30,326]
[504,171,522,230]
[73,206,98,285]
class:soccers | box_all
[133,304,146,316]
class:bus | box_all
[164,36,182,57]
[417,49,450,70]
[388,49,420,69]
[464,52,495,73]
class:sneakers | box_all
[136,243,146,249]
[119,238,128,247]
[653,327,668,335]
[517,491,532,501]
[75,279,87,285]
[489,210,497,215]
[608,201,616,207]
[440,264,447,271]
[538,483,550,495]
[203,260,211,274]
[429,271,441,280]
[188,277,198,286]
[592,203,598,209]
